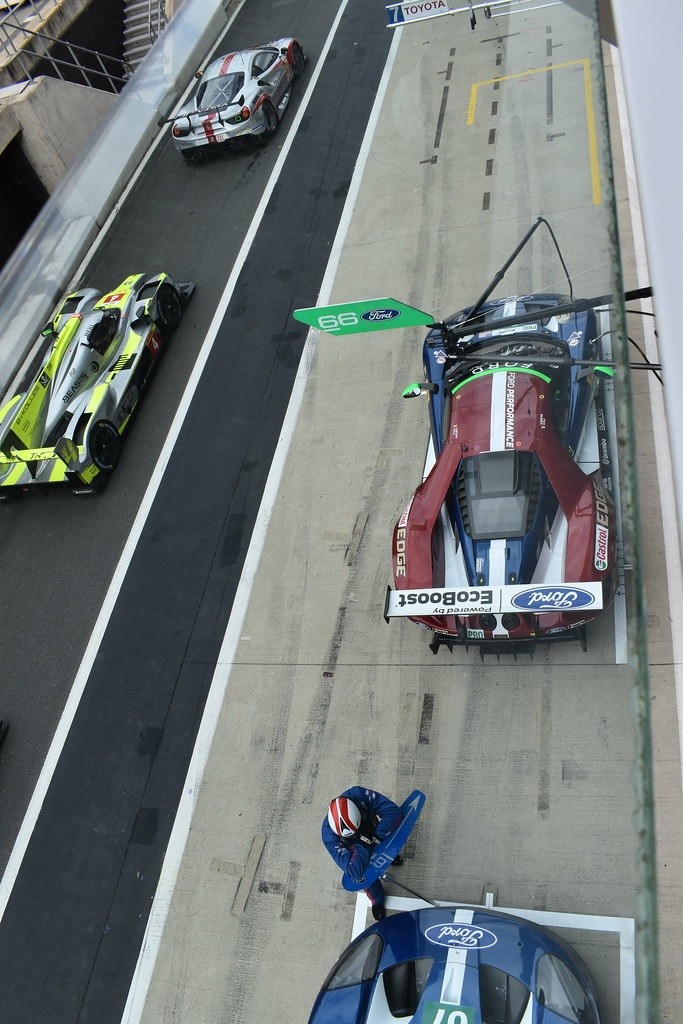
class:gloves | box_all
[360,823,373,847]
[373,834,383,847]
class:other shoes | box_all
[372,903,386,920]
[390,855,403,866]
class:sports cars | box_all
[158,36,309,166]
[0,270,197,501]
[383,291,613,663]
[306,906,605,1024]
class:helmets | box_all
[327,797,362,838]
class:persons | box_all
[322,785,403,924]
